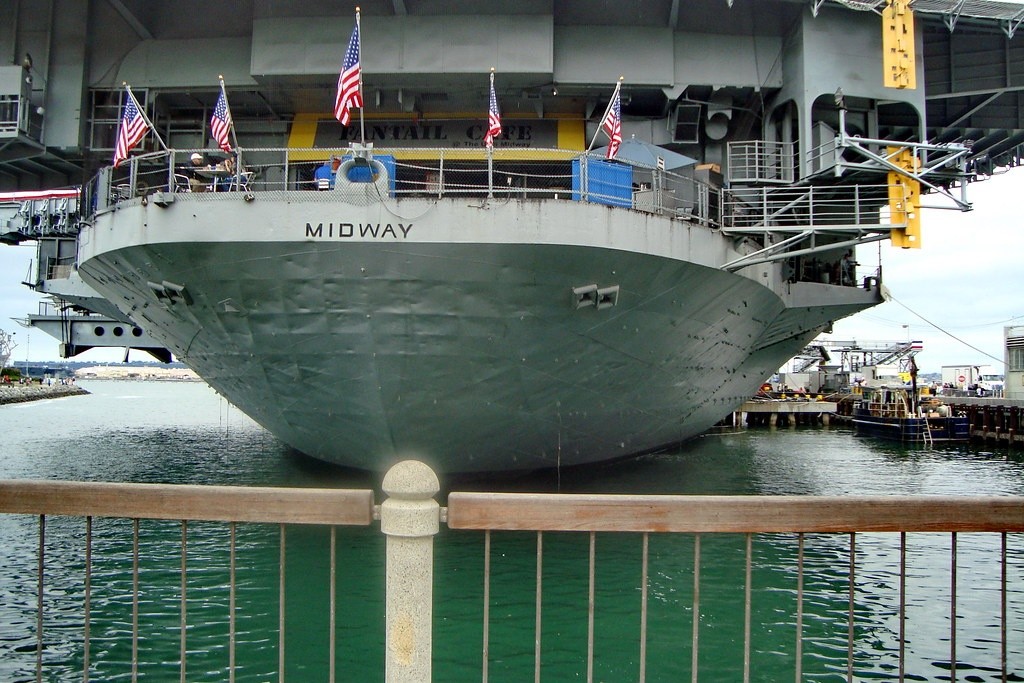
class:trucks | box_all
[942,364,1004,391]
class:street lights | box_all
[902,324,910,345]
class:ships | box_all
[1,2,1024,479]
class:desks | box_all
[194,169,226,193]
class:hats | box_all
[231,153,236,157]
[191,153,202,159]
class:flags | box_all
[112,83,151,170]
[596,86,624,163]
[331,13,365,129]
[210,79,234,152]
[480,73,502,148]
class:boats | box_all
[849,355,972,448]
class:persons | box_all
[179,152,203,192]
[817,384,826,393]
[931,381,937,396]
[812,256,823,283]
[842,253,850,281]
[944,381,955,389]
[222,151,247,191]
[39,377,50,386]
[784,386,788,392]
[17,376,31,387]
[312,158,341,190]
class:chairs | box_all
[229,172,257,194]
[173,174,192,194]
[318,178,335,191]
